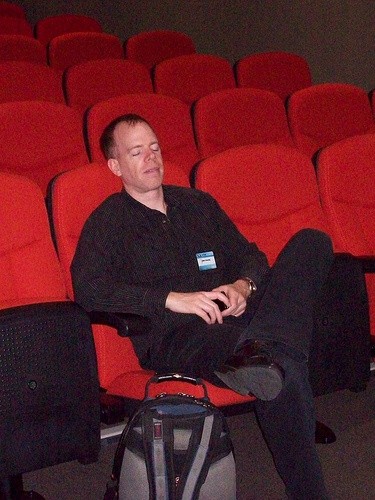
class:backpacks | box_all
[104,371,238,500]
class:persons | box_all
[69,114,334,500]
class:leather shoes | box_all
[214,355,282,401]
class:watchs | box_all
[239,277,255,299]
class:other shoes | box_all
[315,421,336,443]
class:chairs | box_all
[0,0,375,500]
